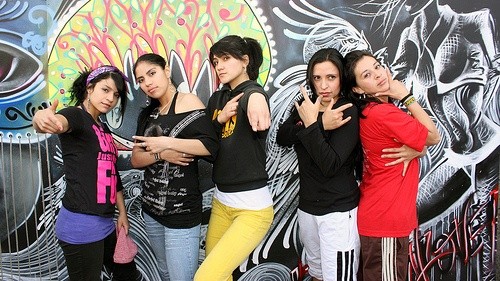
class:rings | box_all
[143,142,146,147]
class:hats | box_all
[113,222,138,264]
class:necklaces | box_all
[80,102,86,112]
[160,95,174,112]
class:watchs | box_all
[155,153,162,162]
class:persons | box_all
[344,50,440,281]
[276,48,362,281]
[32,35,274,281]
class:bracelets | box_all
[400,93,416,108]
